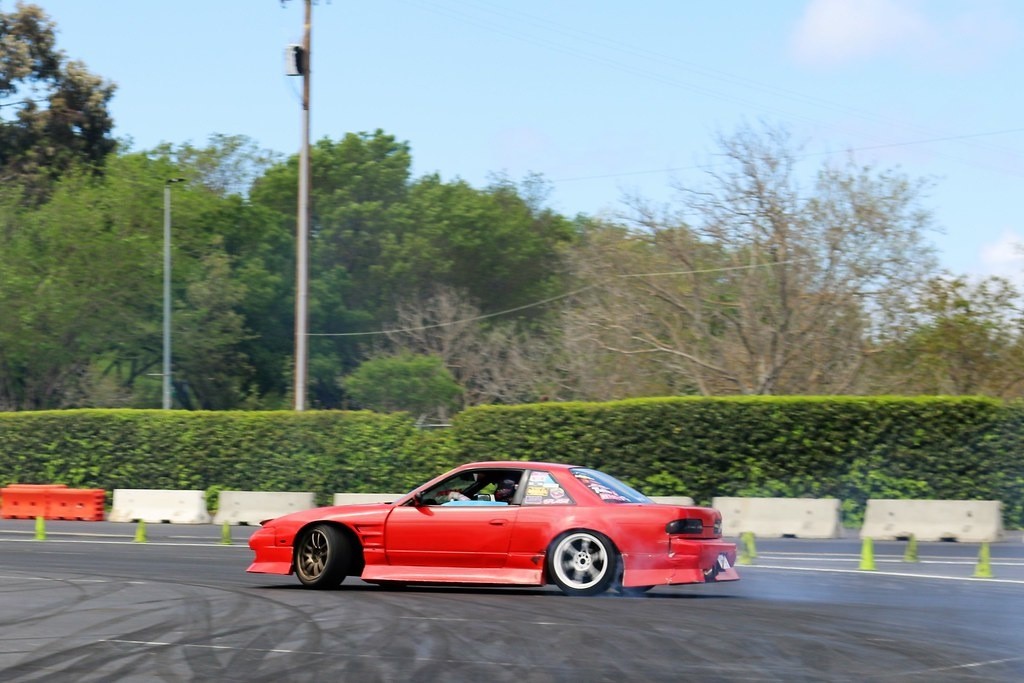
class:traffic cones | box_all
[852,534,879,572]
[212,520,236,546]
[737,531,757,566]
[130,519,150,543]
[967,540,998,580]
[902,533,920,563]
[30,516,49,540]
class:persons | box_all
[448,479,516,505]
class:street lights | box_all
[162,175,188,410]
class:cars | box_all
[248,460,743,596]
